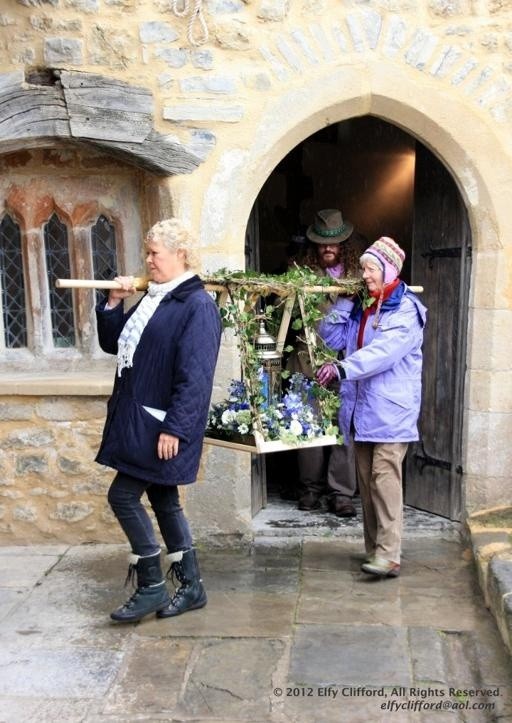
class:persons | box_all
[285,208,364,516]
[94,218,223,623]
[315,235,429,577]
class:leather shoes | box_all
[298,489,357,518]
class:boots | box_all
[111,547,207,621]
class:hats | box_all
[364,236,406,286]
[306,209,353,244]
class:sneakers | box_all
[362,558,400,577]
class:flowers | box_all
[207,372,333,446]
